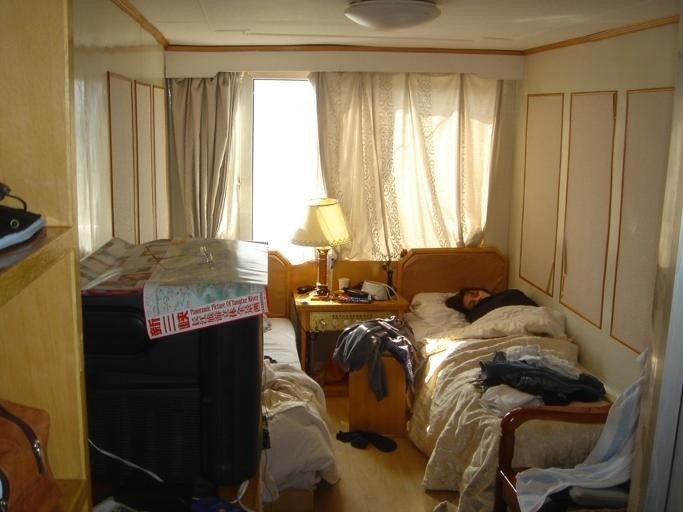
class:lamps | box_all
[343,0,441,32]
[290,198,353,301]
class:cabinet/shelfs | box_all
[0,0,93,512]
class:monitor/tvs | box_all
[82,295,258,512]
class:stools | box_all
[348,349,408,437]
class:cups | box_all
[338,278,350,290]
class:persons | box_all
[444,287,565,397]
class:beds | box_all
[261,251,315,512]
[397,247,619,512]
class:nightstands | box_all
[291,289,409,399]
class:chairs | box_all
[494,403,629,512]
